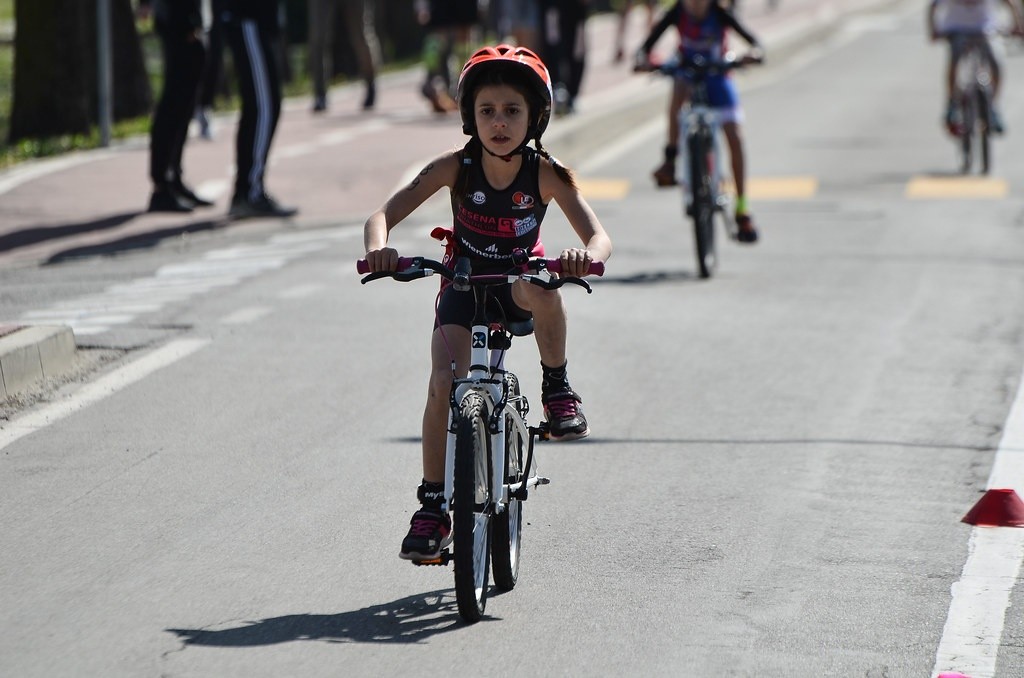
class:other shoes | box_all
[736,218,758,242]
[313,95,325,111]
[175,182,214,207]
[989,111,1003,134]
[150,191,193,213]
[948,108,964,135]
[364,92,375,108]
[654,159,677,188]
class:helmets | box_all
[458,45,553,140]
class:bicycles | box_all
[629,52,769,283]
[923,25,1023,177]
[354,249,605,626]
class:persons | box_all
[362,43,614,563]
[311,0,378,111]
[497,1,655,117]
[630,0,766,243]
[413,0,489,114]
[928,0,1023,139]
[135,0,225,214]
[218,0,299,220]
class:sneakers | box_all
[230,195,298,217]
[399,506,455,559]
[541,388,590,441]
